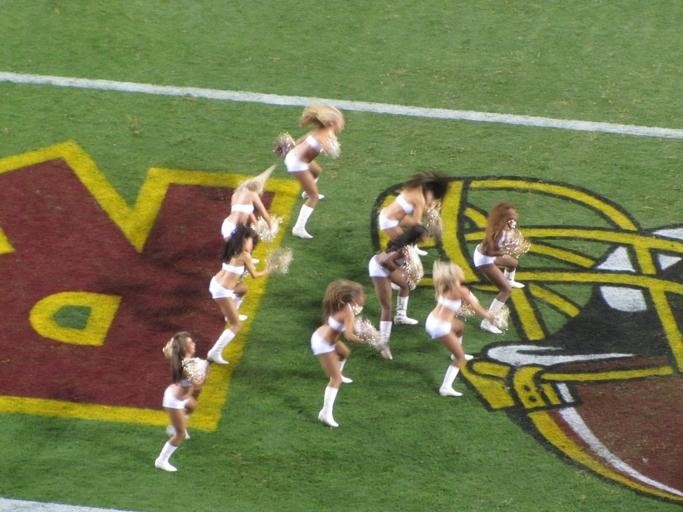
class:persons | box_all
[368,226,432,343]
[278,105,344,240]
[221,181,274,264]
[379,172,451,289]
[310,280,369,429]
[207,223,273,365]
[424,261,506,397]
[474,202,525,333]
[154,332,207,473]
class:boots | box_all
[504,269,525,288]
[319,386,339,427]
[292,206,314,239]
[450,335,473,361]
[168,412,190,440]
[302,176,324,200]
[439,364,463,398]
[155,443,178,472]
[413,243,428,257]
[393,294,418,325]
[391,283,402,290]
[207,328,236,366]
[481,297,504,334]
[378,321,394,360]
[339,360,353,383]
[225,296,249,321]
